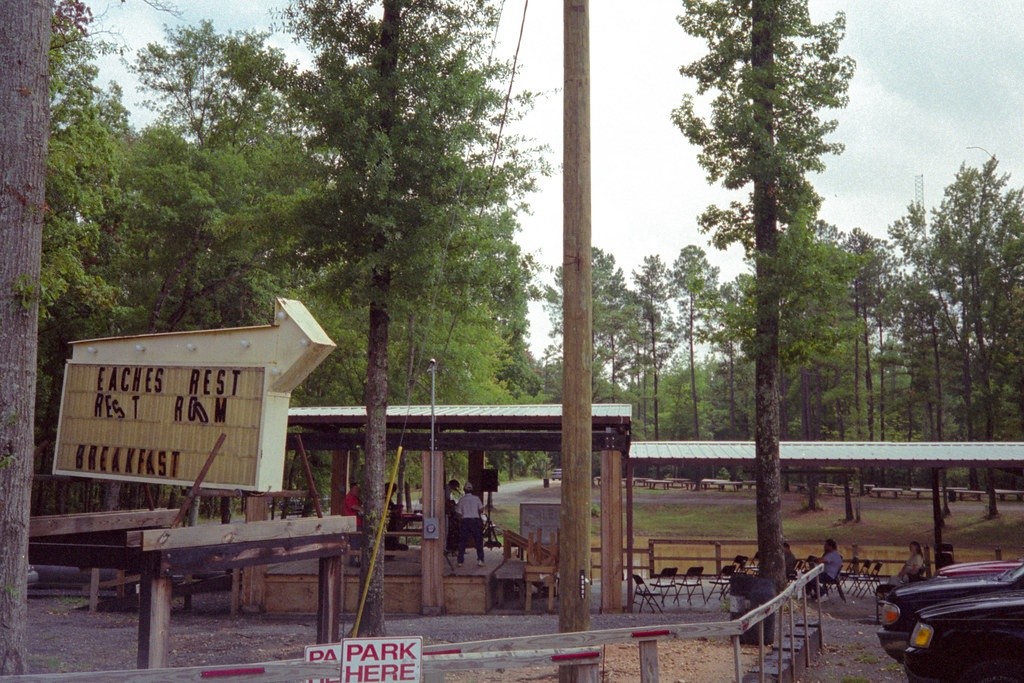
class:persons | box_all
[784,542,796,568]
[808,539,843,598]
[344,483,364,566]
[458,482,485,567]
[889,541,923,583]
[444,480,460,556]
[386,483,409,560]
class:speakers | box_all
[482,469,498,492]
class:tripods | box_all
[482,492,500,550]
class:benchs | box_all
[621,478,757,492]
[494,558,527,610]
[795,483,1024,501]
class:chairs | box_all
[632,552,760,614]
[875,584,900,625]
[793,554,883,600]
[910,563,926,584]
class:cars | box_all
[876,561,1024,663]
[552,468,565,480]
[904,589,1024,683]
[936,559,1023,579]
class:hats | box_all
[464,482,473,491]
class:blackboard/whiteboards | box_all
[519,504,561,545]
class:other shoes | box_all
[478,560,486,566]
[457,561,464,566]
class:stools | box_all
[523,526,557,615]
